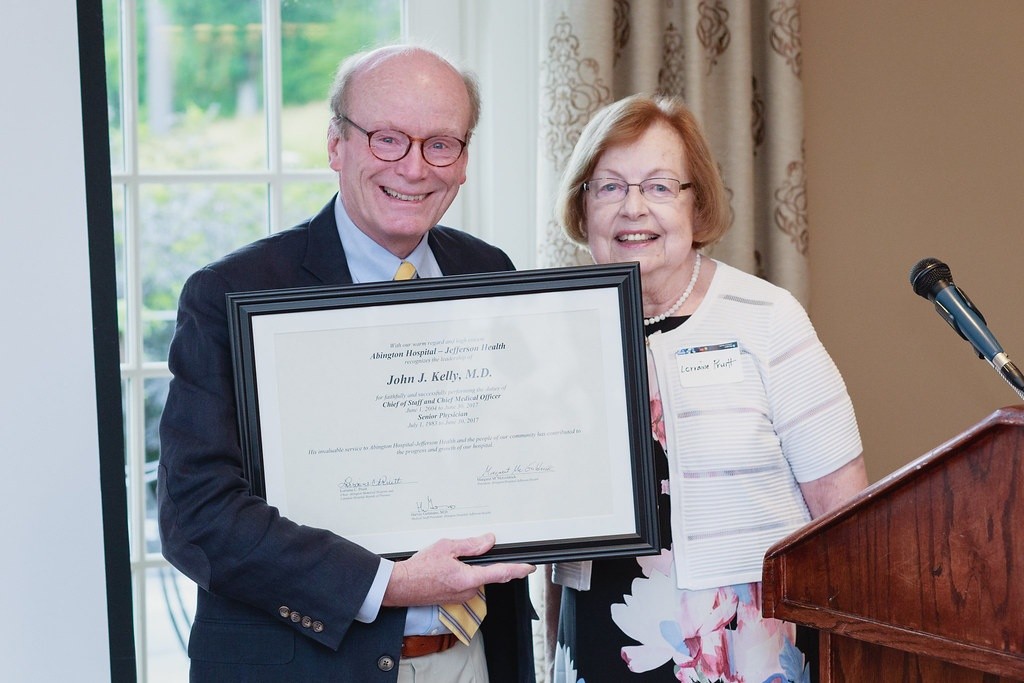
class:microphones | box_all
[910,257,1024,392]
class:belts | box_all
[401,634,459,659]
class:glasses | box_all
[583,178,694,205]
[339,113,469,168]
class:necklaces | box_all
[644,252,700,326]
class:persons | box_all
[546,94,868,683]
[158,46,538,683]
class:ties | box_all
[392,261,488,646]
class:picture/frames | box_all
[223,261,662,567]
